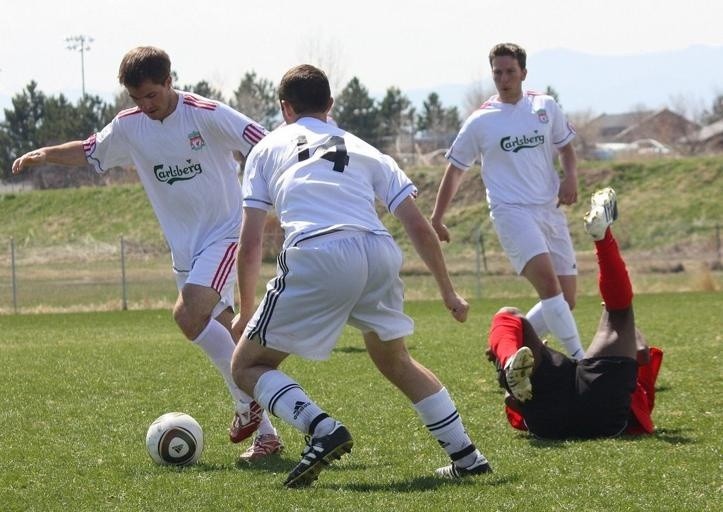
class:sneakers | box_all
[435,449,493,479]
[503,347,534,403]
[584,187,618,240]
[229,400,264,442]
[229,427,283,462]
[283,421,353,487]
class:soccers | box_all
[145,411,203,466]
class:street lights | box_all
[65,34,95,98]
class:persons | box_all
[228,62,496,488]
[484,185,664,441]
[429,44,584,361]
[12,45,285,462]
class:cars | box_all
[596,138,670,154]
[397,148,450,164]
